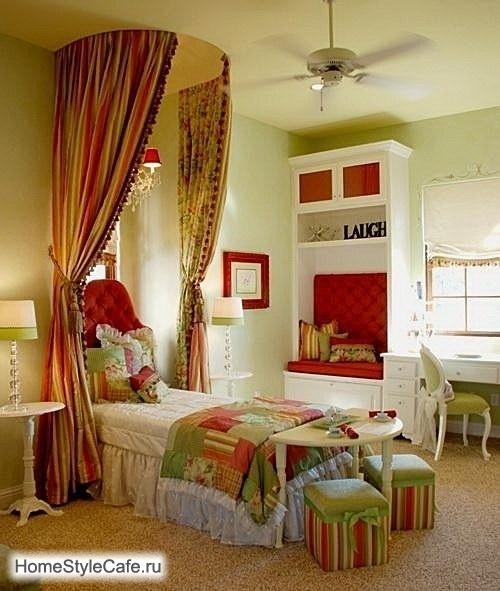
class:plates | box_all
[308,413,360,429]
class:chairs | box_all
[420,345,492,461]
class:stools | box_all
[364,454,436,530]
[304,479,391,572]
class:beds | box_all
[209,373,254,396]
[82,279,373,547]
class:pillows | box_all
[296,319,376,362]
[85,323,171,404]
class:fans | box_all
[250,0,433,96]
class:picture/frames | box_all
[224,250,270,310]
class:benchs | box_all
[287,271,409,381]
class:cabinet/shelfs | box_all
[288,140,414,248]
[283,370,383,410]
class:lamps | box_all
[0,300,39,412]
[311,72,344,91]
[211,297,244,375]
[126,148,162,212]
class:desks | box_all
[379,352,500,438]
[269,407,403,548]
